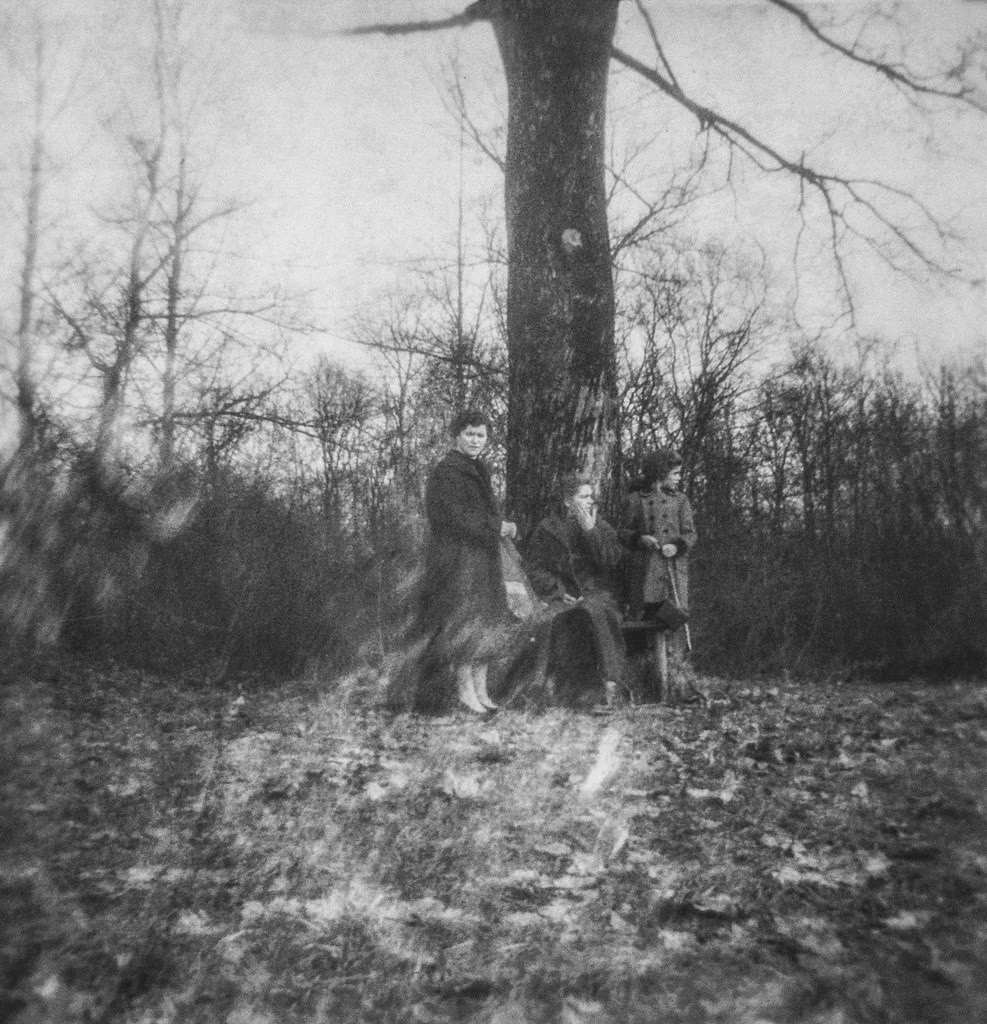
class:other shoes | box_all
[457,699,501,721]
[593,682,617,714]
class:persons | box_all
[618,448,708,705]
[524,477,625,714]
[426,409,517,722]
